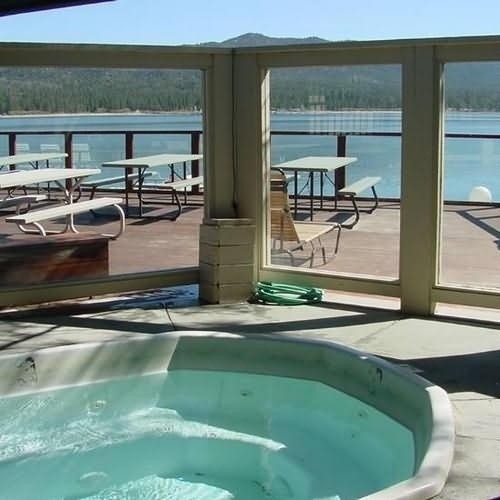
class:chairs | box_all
[267,167,340,267]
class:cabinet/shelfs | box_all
[199,218,257,306]
